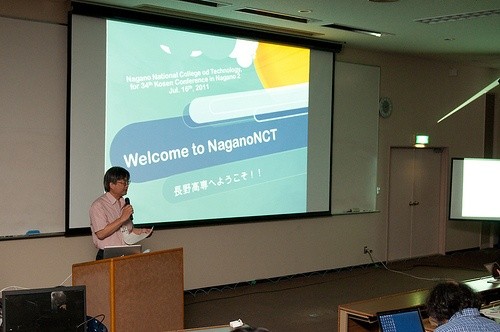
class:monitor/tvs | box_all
[376,306,426,332]
[103,245,143,259]
[2,285,88,332]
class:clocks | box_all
[380,96,392,119]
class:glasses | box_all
[113,181,131,186]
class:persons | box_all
[89,166,154,250]
[424,280,500,332]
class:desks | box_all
[338,275,500,332]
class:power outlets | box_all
[364,246,368,254]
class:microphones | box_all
[125,198,133,220]
